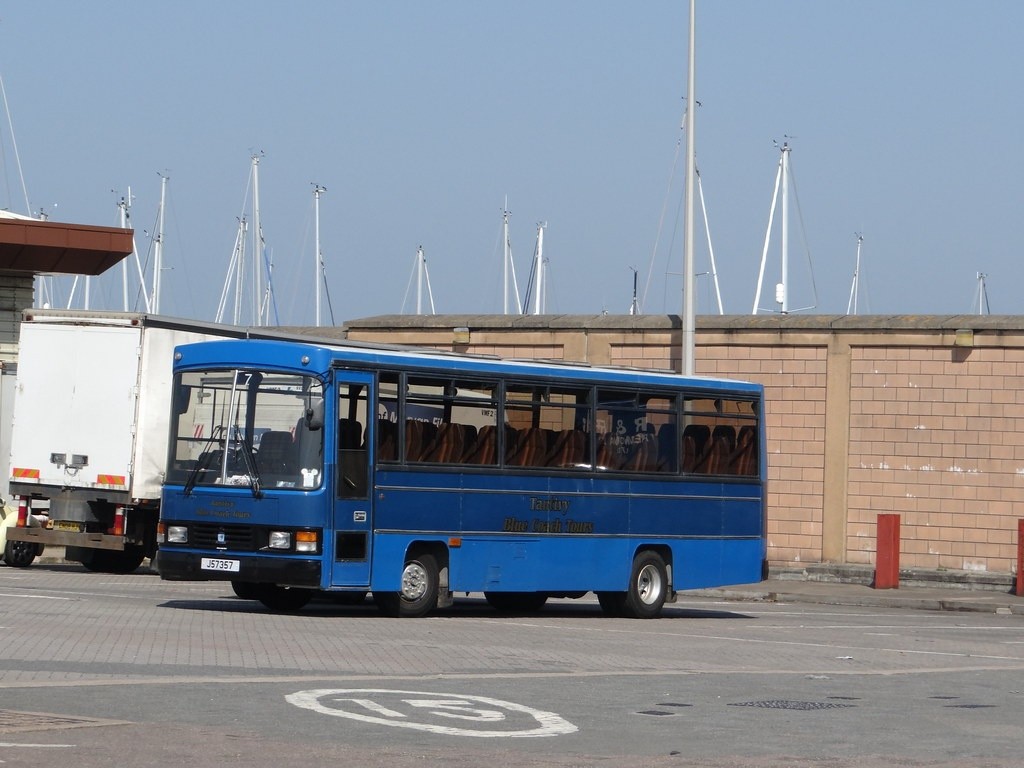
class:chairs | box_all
[335,420,758,477]
[253,431,293,462]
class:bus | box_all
[152,337,770,618]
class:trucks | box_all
[1,307,511,575]
